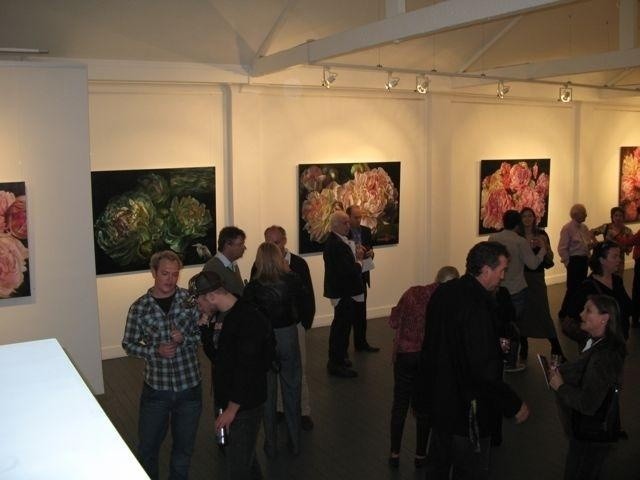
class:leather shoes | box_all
[365,344,380,352]
[344,358,352,367]
[328,361,357,376]
[277,411,285,424]
[389,456,399,467]
[415,456,428,467]
[302,416,313,431]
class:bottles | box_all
[244,279,248,286]
[214,408,229,449]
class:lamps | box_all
[312,55,587,108]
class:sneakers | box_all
[504,364,525,373]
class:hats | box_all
[188,270,222,304]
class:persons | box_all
[121,248,210,479]
[242,241,302,460]
[186,269,280,476]
[550,294,638,478]
[323,204,382,376]
[249,225,316,432]
[202,226,248,297]
[386,207,568,480]
[556,203,640,337]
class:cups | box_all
[500,337,511,353]
[548,354,563,381]
[529,239,538,248]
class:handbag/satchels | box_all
[561,315,592,347]
[561,384,629,443]
